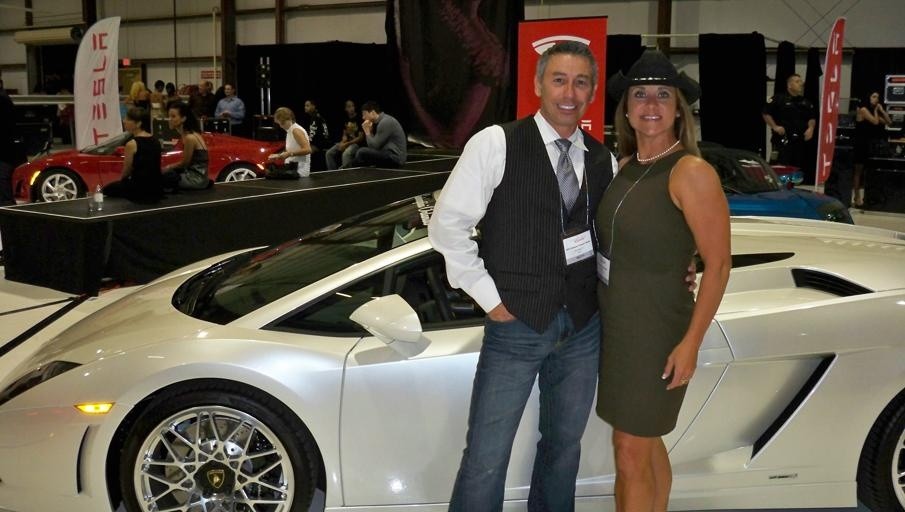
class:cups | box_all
[86,193,96,211]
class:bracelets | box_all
[290,151,293,156]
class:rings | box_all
[680,379,688,384]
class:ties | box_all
[555,138,579,214]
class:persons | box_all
[762,75,818,167]
[354,101,406,168]
[305,100,329,170]
[268,107,312,176]
[120,80,246,134]
[99,107,162,199]
[325,100,365,170]
[851,88,892,207]
[162,102,209,189]
[427,41,697,512]
[593,50,732,512]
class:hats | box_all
[609,49,702,105]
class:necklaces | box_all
[636,140,681,162]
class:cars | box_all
[697,136,856,228]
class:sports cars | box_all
[10,128,286,208]
[0,186,904,512]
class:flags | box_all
[74,16,123,148]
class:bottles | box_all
[94,185,103,211]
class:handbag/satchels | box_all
[267,162,300,180]
